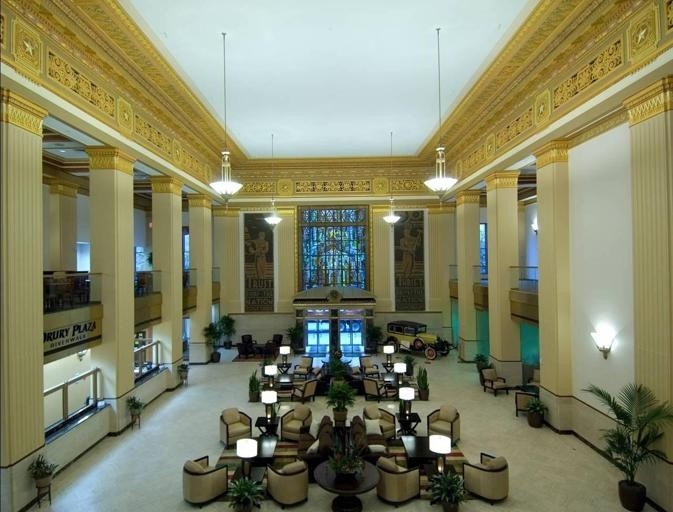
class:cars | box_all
[381,320,450,359]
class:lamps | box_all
[210,34,243,215]
[382,132,402,228]
[590,332,616,360]
[264,135,283,230]
[423,29,458,209]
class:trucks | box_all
[307,309,362,332]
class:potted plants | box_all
[204,324,221,362]
[217,315,236,349]
[177,364,189,378]
[126,396,145,415]
[580,383,673,512]
[427,471,470,512]
[522,397,549,428]
[224,476,264,512]
[27,455,60,488]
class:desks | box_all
[315,459,382,512]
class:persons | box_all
[248,232,269,279]
[400,228,421,279]
